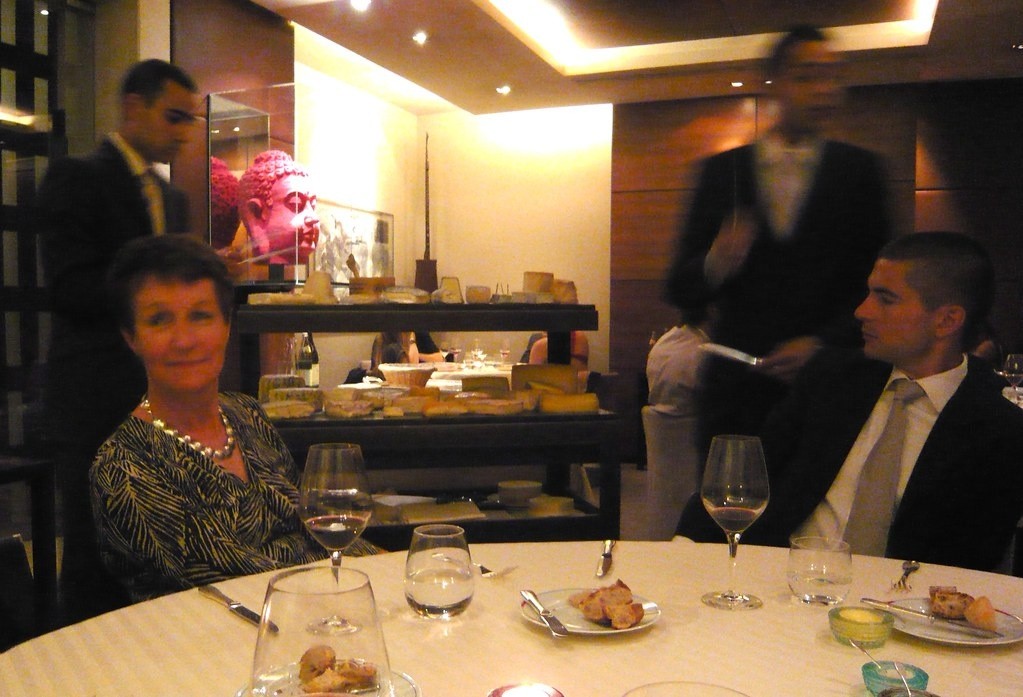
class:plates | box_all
[886,598,1023,647]
[234,658,423,697]
[621,681,750,697]
[521,588,661,635]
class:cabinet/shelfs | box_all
[233,303,638,552]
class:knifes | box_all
[859,598,1004,638]
[520,590,569,639]
[596,539,617,578]
[197,584,279,635]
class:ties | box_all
[841,376,929,559]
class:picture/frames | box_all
[314,198,395,286]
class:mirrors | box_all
[208,91,270,249]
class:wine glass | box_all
[1004,353,1023,395]
[440,338,462,365]
[500,342,510,367]
[701,435,771,611]
[299,443,374,635]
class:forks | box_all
[891,560,920,595]
[431,552,517,580]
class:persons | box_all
[238,150,320,265]
[522,329,590,375]
[211,155,242,250]
[36,57,202,613]
[670,229,1023,576]
[85,232,394,602]
[370,325,449,381]
[664,24,894,540]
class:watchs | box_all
[408,338,416,344]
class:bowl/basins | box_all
[497,481,576,519]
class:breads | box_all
[928,585,996,632]
[298,646,377,696]
[579,580,645,628]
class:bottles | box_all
[297,332,319,389]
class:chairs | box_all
[0,441,132,653]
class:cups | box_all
[861,660,942,697]
[402,524,474,621]
[786,537,853,609]
[248,565,396,697]
[828,606,895,648]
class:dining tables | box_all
[0,540,1023,697]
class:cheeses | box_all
[247,272,579,305]
[254,363,601,419]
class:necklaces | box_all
[139,394,235,459]
